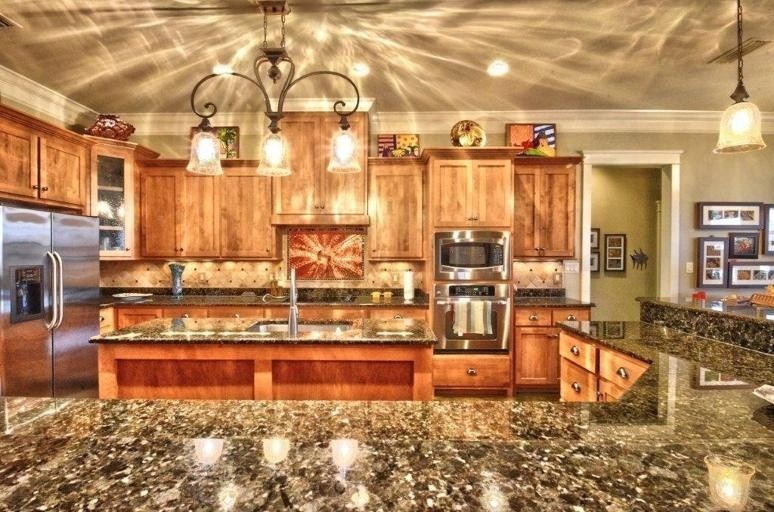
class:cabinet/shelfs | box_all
[137,159,222,261]
[162,307,208,318]
[270,112,369,225]
[433,354,513,389]
[208,306,263,318]
[116,305,162,329]
[368,158,426,260]
[84,135,161,262]
[513,307,590,390]
[421,148,525,229]
[0,101,96,215]
[221,160,282,262]
[513,157,584,260]
[99,305,116,334]
[556,329,598,402]
[598,345,652,403]
[369,309,428,320]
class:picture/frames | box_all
[694,365,755,390]
[603,234,627,272]
[591,251,600,273]
[591,227,600,251]
[728,260,774,289]
[504,122,557,158]
[697,237,730,289]
[762,204,774,255]
[727,232,760,260]
[189,126,240,159]
[697,201,765,230]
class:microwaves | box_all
[434,231,510,280]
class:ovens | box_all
[433,282,510,350]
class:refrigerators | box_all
[0,204,97,400]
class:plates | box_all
[111,292,153,301]
[449,119,486,148]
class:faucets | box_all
[288,267,299,336]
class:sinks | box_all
[245,319,354,333]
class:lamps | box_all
[184,0,363,179]
[713,1,766,155]
